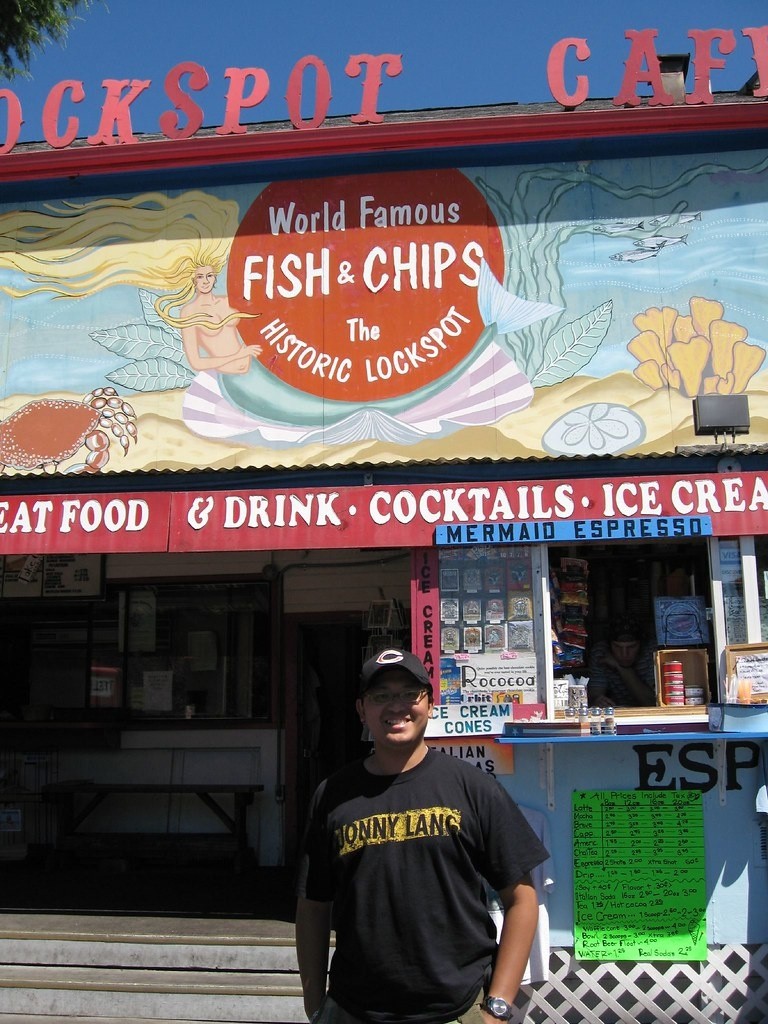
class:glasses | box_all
[366,688,430,705]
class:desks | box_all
[41,779,265,876]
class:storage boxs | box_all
[654,648,712,707]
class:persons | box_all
[585,620,654,708]
[296,647,551,1024]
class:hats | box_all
[359,647,433,694]
[609,612,642,642]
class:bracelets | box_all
[310,1010,318,1024]
[483,995,514,1020]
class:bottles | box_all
[591,706,602,735]
[577,706,589,722]
[564,707,576,722]
[185,704,195,719]
[604,706,615,734]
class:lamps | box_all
[692,393,749,443]
